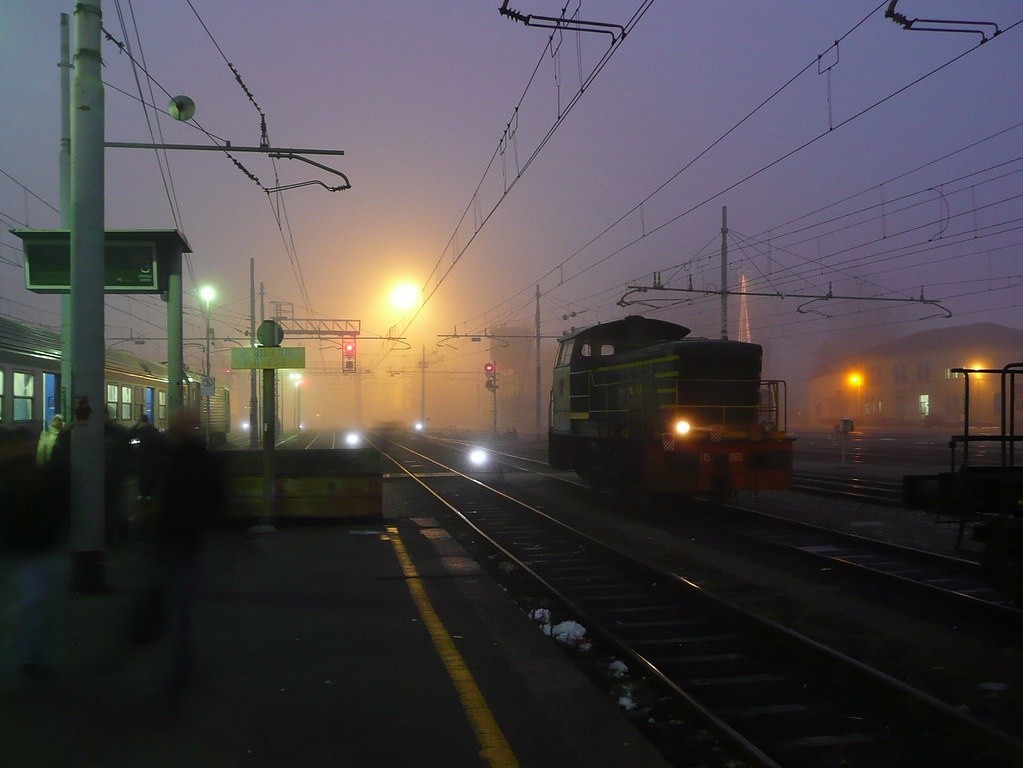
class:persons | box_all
[38,413,65,464]
[127,405,220,639]
[131,415,160,438]
[51,402,132,593]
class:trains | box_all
[1,316,232,464]
[544,315,799,500]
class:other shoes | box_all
[137,491,153,504]
